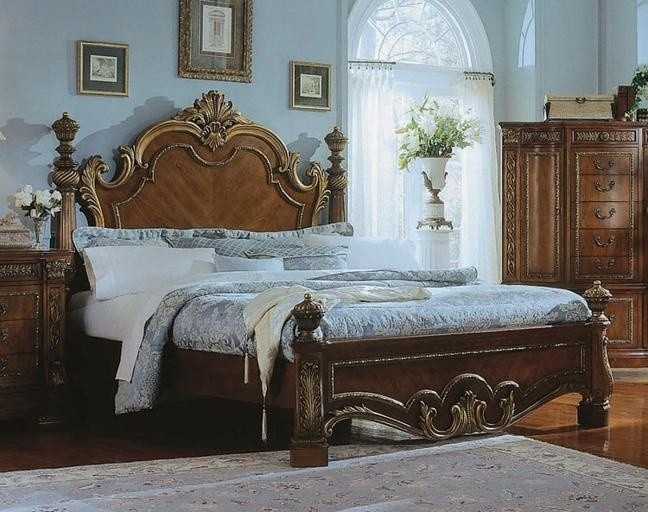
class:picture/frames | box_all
[179,0,254,83]
[291,60,332,111]
[77,41,129,96]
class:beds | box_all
[51,89,614,468]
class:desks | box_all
[414,229,460,268]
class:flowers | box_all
[395,89,487,173]
[14,185,62,217]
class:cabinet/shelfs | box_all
[498,122,648,367]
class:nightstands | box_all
[1,250,76,421]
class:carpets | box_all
[0,432,648,512]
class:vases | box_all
[32,217,49,250]
[422,158,449,218]
